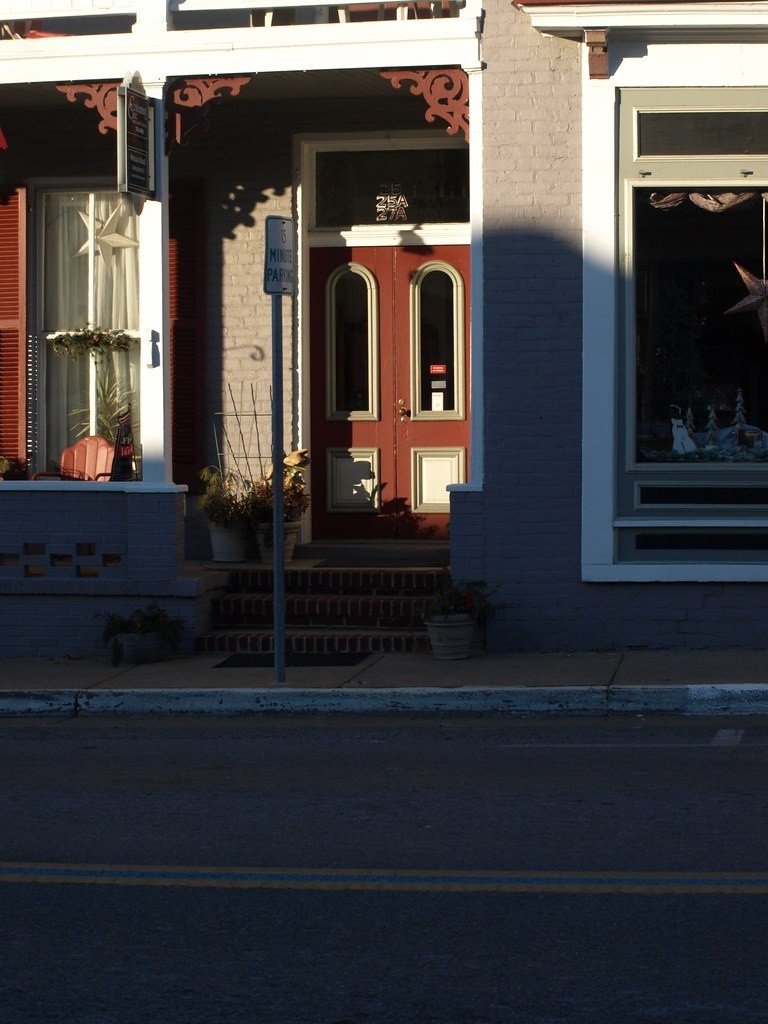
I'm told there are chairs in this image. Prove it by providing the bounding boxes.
[31,435,115,482]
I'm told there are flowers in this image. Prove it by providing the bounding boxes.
[416,579,522,626]
[46,324,141,375]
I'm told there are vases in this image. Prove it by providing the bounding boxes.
[425,612,472,660]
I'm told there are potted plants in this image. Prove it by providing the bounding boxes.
[195,464,312,564]
